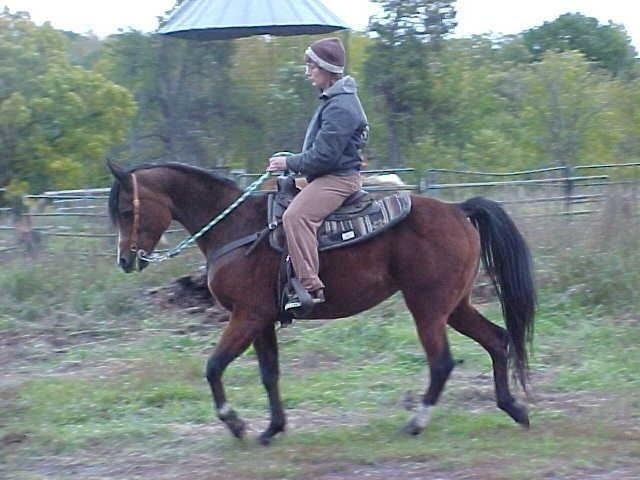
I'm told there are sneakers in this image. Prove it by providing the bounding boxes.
[285,287,327,311]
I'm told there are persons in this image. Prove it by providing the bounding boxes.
[267,38,369,311]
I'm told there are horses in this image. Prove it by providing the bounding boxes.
[104,159,538,449]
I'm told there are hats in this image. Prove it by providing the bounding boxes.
[304,37,346,74]
